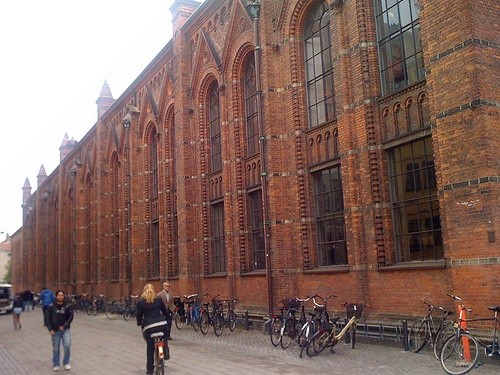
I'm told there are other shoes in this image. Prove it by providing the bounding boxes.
[53,365,60,371]
[168,336,172,340]
[164,351,170,359]
[64,364,71,370]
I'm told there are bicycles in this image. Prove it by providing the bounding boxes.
[137,322,168,375]
[173,293,237,337]
[269,294,369,357]
[407,301,458,362]
[63,294,139,321]
[437,294,500,375]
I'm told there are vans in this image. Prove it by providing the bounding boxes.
[0,284,14,313]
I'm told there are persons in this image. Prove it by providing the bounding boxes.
[136,284,171,375]
[12,295,23,330]
[44,290,74,370]
[157,281,174,341]
[39,287,54,325]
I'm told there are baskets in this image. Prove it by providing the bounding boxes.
[284,297,301,309]
[347,303,363,319]
